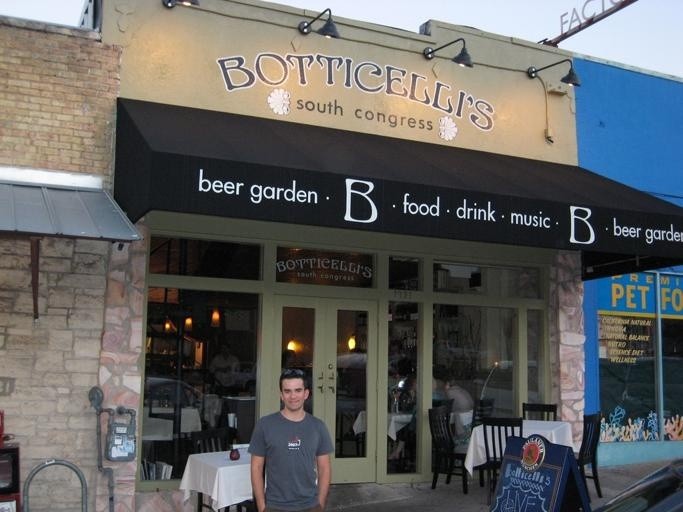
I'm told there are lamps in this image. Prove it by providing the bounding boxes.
[162,290,223,334]
[529,59,581,85]
[163,1,200,9]
[298,9,342,38]
[423,38,474,68]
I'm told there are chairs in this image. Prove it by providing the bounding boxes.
[572,412,603,503]
[427,407,484,494]
[481,416,524,506]
[192,426,236,455]
[522,402,558,420]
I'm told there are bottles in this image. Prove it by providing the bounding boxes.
[230,449,240,460]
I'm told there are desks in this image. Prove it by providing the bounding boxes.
[465,420,576,478]
[180,449,320,510]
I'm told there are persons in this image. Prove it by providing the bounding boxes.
[386,377,443,461]
[440,374,475,438]
[249,367,335,510]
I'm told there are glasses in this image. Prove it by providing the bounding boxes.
[281,368,303,376]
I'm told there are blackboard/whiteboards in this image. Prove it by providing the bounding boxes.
[489,436,572,512]
